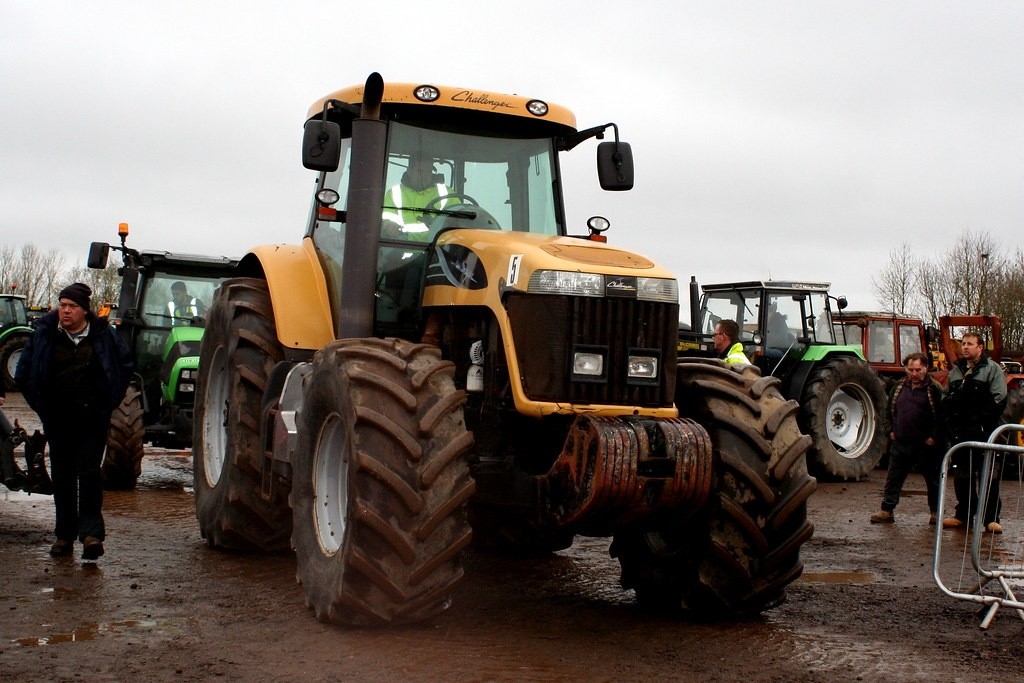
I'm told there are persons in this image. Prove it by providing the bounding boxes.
[16,283,128,561]
[767,302,788,347]
[162,281,208,327]
[712,319,751,364]
[946,332,1008,534]
[381,149,463,280]
[869,353,946,526]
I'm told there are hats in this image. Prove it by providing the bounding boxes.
[58,283,92,313]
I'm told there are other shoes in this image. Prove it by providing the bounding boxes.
[50,538,75,557]
[81,535,104,561]
[930,512,937,524]
[986,521,1003,534]
[870,509,895,524]
[943,516,973,529]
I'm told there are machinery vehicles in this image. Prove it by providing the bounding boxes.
[0,282,53,393]
[689,275,889,484]
[192,70,819,629]
[818,312,1023,482]
[88,222,242,492]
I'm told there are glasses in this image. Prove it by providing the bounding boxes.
[710,333,729,340]
